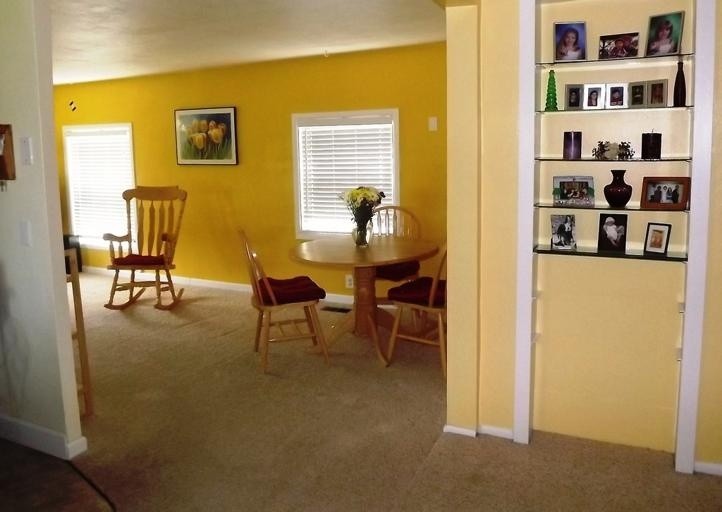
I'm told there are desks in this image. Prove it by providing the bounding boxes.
[287,236,440,368]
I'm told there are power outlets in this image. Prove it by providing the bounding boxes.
[345,274,354,289]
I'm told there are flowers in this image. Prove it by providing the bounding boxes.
[592,140,637,163]
[338,185,386,244]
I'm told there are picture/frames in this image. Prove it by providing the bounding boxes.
[552,173,597,208]
[642,221,673,259]
[173,105,240,167]
[641,175,692,212]
[552,9,688,109]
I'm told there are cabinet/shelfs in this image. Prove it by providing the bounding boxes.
[532,47,695,262]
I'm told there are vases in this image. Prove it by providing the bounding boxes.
[351,223,373,248]
[604,169,633,210]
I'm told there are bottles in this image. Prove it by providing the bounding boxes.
[544,68,559,111]
[673,57,687,106]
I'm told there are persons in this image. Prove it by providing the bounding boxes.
[556,20,675,110]
[554,181,680,255]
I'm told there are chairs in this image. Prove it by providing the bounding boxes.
[236,228,332,372]
[103,184,188,311]
[370,204,423,330]
[386,239,447,379]
